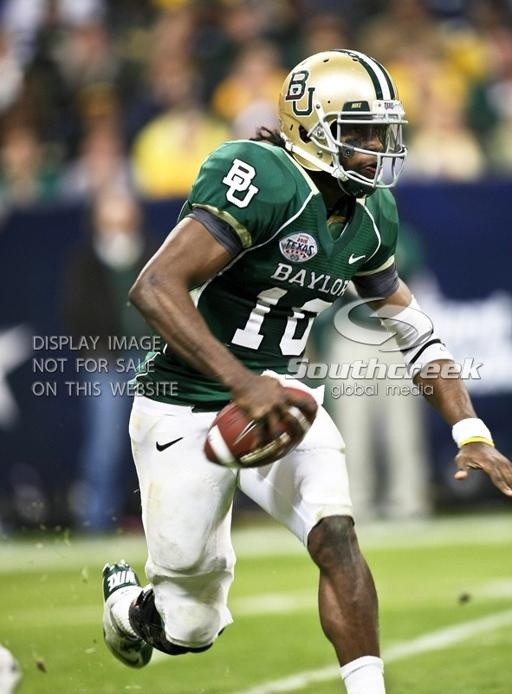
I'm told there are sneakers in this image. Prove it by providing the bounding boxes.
[100,559,153,670]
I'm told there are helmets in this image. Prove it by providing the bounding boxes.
[279,48,407,173]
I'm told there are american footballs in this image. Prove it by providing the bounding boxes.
[204,387,317,468]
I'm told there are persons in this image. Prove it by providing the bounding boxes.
[103,49,512,694]
